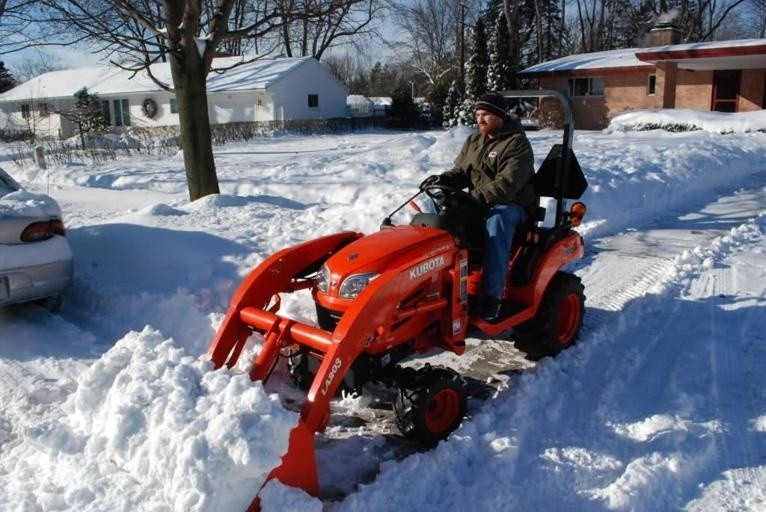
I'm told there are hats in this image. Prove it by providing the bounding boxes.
[475,92,508,118]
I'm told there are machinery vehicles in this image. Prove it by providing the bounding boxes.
[197,85,591,509]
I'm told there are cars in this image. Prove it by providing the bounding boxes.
[0,166,77,317]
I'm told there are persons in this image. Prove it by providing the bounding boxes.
[416,92,539,323]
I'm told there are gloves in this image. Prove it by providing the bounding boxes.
[420,175,444,191]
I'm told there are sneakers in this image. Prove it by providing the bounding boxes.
[480,297,503,322]
